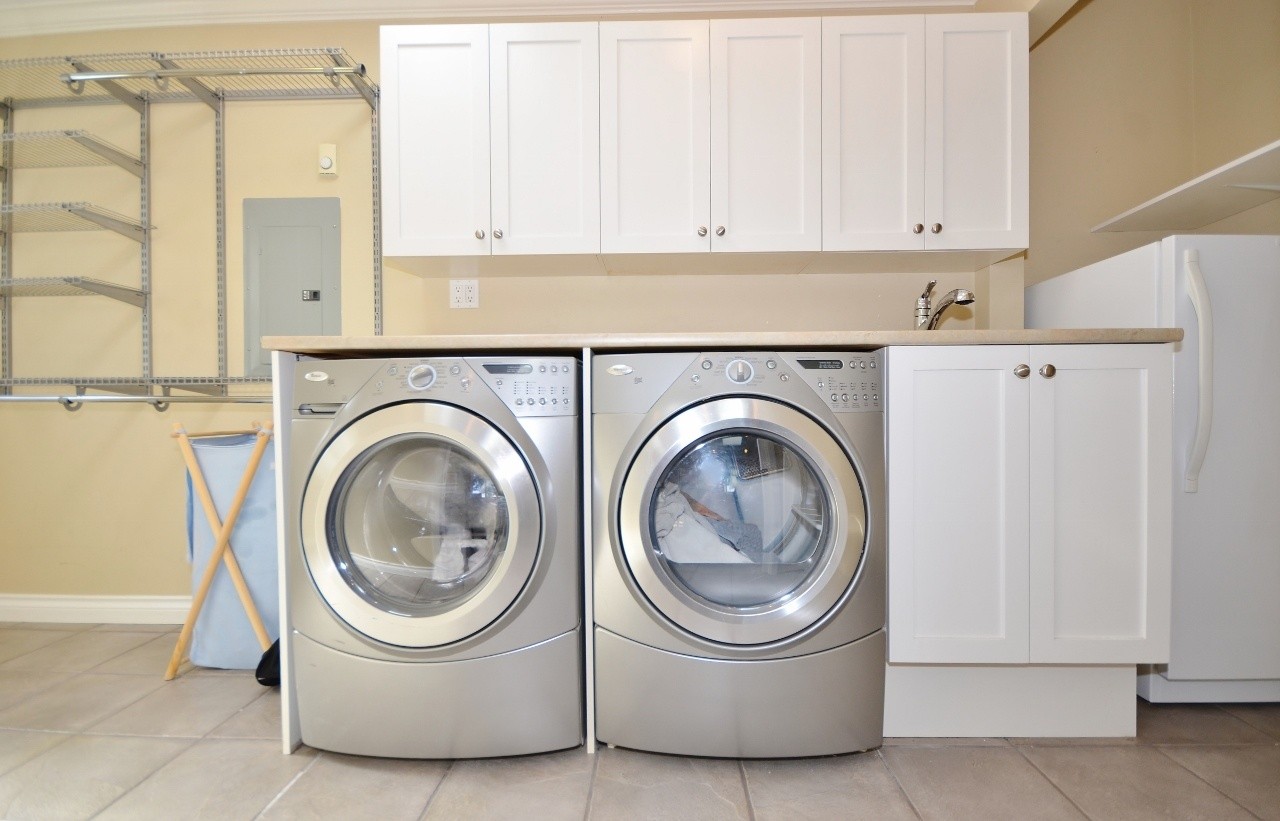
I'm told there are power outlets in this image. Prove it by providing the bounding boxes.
[449,280,479,308]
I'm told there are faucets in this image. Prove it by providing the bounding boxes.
[914,279,976,330]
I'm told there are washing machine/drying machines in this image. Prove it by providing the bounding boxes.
[258,330,891,760]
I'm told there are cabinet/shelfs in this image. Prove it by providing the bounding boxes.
[885,346,1174,741]
[0,41,387,413]
[374,1,1037,274]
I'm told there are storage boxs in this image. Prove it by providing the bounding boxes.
[163,433,281,683]
